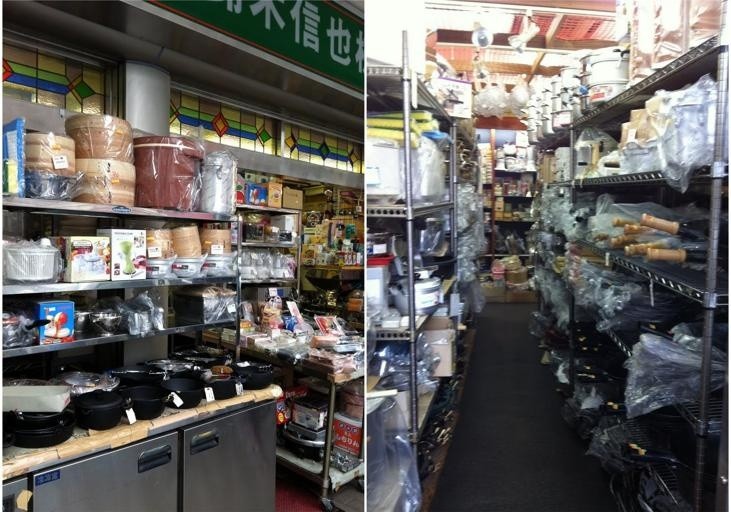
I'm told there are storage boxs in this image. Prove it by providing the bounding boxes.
[257,181,304,209]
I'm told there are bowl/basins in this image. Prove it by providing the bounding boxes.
[74,311,124,337]
[147,257,233,279]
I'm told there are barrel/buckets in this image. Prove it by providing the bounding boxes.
[166,225,201,257]
[200,222,233,257]
[147,227,175,260]
[65,115,134,164]
[22,128,79,178]
[71,158,134,206]
[131,136,205,208]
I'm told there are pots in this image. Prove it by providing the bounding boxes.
[518,45,630,146]
[389,273,442,314]
[2,346,275,447]
[3,310,51,349]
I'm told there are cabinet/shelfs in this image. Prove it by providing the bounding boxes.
[367,1,727,512]
[2,192,365,511]
